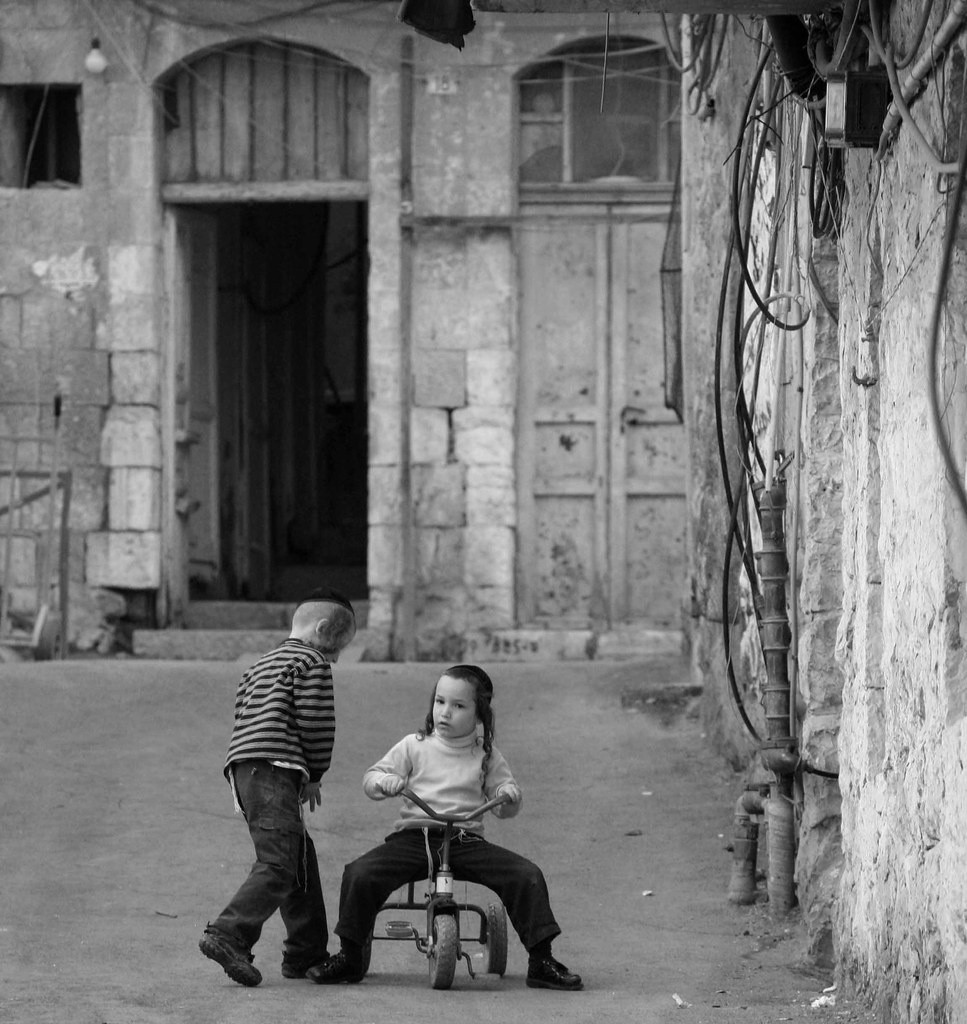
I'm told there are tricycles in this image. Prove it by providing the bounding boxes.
[339,787,513,992]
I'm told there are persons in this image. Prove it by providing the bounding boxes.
[306,665,584,990]
[199,584,355,987]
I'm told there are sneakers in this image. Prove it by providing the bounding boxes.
[525,956,583,992]
[199,931,263,987]
[306,951,364,985]
[281,946,331,978]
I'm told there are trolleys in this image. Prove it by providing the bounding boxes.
[0,393,76,663]
